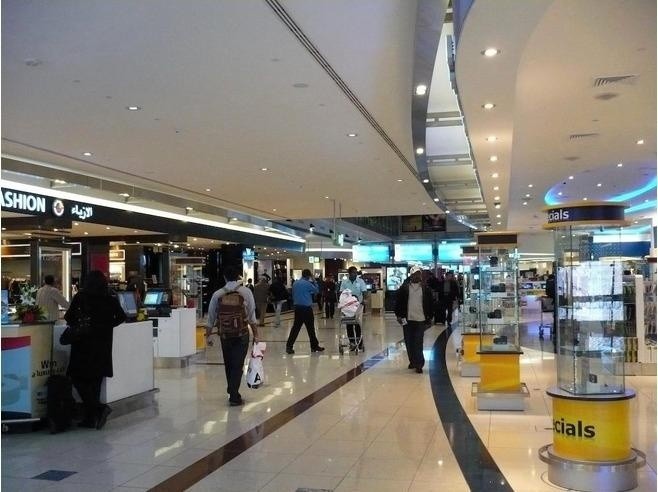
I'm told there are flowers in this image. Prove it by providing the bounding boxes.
[7,278,50,320]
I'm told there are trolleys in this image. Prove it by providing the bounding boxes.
[336,295,366,355]
[538,295,554,338]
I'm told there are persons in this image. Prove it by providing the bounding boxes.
[4,275,22,304]
[314,274,339,319]
[546,274,554,299]
[61,270,126,430]
[393,266,434,374]
[246,276,290,326]
[421,270,464,327]
[339,266,367,351]
[203,266,260,408]
[285,269,325,354]
[35,275,70,324]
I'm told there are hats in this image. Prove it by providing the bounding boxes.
[410,266,422,277]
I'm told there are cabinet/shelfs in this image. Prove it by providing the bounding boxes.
[540,200,640,492]
[460,244,497,377]
[470,233,529,411]
[170,254,206,350]
[621,273,645,364]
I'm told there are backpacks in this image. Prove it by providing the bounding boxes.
[215,285,249,339]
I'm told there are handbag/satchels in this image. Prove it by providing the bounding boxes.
[246,341,267,390]
[59,290,93,346]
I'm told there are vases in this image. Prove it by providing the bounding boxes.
[22,310,38,323]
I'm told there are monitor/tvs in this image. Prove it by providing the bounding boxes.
[142,291,164,306]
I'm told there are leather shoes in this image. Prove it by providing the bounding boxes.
[311,346,325,352]
[286,348,294,354]
[77,403,113,430]
[408,363,415,369]
[416,367,423,373]
[229,392,243,406]
